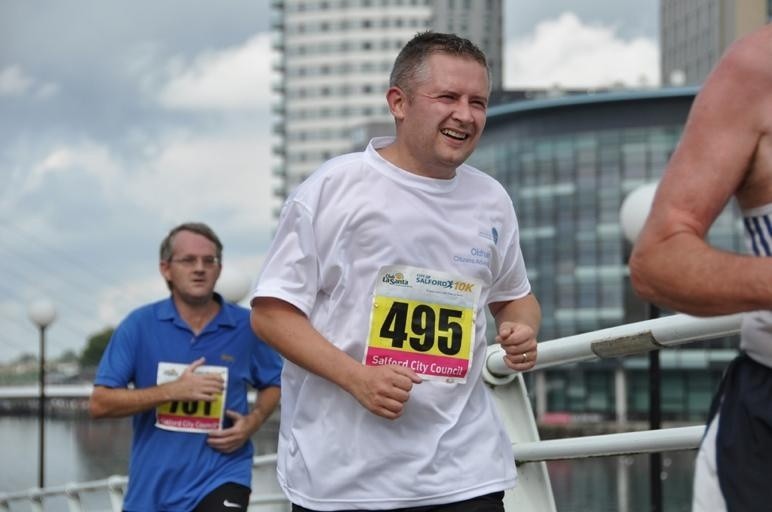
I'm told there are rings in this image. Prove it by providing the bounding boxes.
[523,352,528,362]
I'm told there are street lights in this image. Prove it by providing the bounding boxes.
[620,181,662,512]
[26,295,58,489]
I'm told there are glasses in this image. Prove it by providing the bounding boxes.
[168,255,223,268]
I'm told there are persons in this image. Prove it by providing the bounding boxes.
[249,31,544,511]
[626,20,772,511]
[89,221,283,512]
